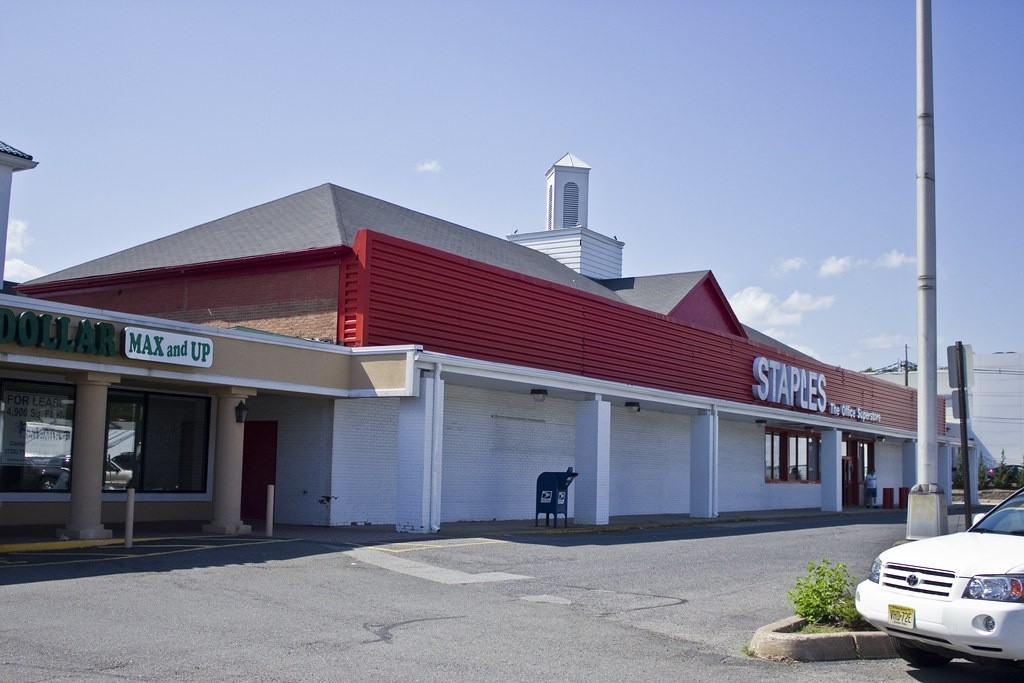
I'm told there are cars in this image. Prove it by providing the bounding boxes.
[38,454,134,492]
[853,485,1023,671]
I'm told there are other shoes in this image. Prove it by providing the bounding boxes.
[867,505,869,508]
[874,506,879,509]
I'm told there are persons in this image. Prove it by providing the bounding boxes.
[864,467,877,509]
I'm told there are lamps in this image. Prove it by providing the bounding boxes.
[842,432,885,442]
[234,399,249,424]
[530,389,548,403]
[624,401,640,412]
[755,420,767,428]
[805,426,815,433]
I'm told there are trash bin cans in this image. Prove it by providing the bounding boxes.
[535,467,579,529]
